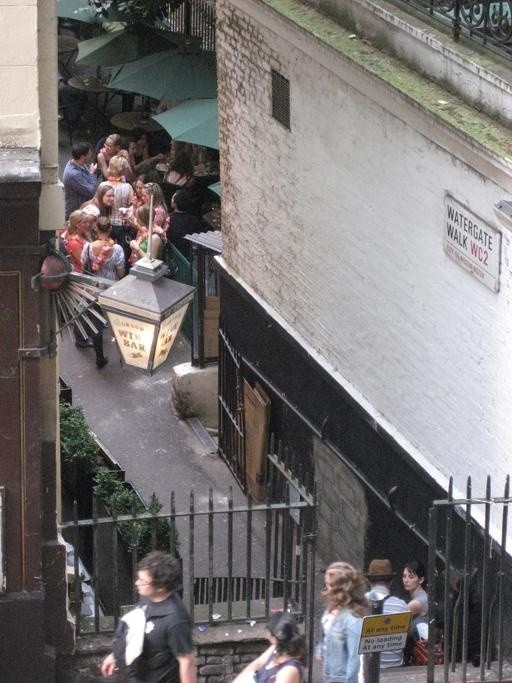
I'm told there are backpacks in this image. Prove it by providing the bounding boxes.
[367,593,393,616]
[112,596,185,683]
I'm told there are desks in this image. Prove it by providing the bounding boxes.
[111,110,165,132]
[58,35,81,75]
[67,73,135,128]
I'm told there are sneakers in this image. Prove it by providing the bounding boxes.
[75,340,108,368]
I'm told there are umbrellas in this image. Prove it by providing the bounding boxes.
[56,0,221,202]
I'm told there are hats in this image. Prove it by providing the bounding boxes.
[363,558,399,578]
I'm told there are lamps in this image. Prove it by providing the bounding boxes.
[31,194,198,376]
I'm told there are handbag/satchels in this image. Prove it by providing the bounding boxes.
[410,638,445,666]
[82,243,93,276]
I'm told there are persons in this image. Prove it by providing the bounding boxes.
[357,558,412,683]
[100,549,197,683]
[402,558,431,661]
[60,126,218,370]
[232,612,304,682]
[313,561,368,682]
